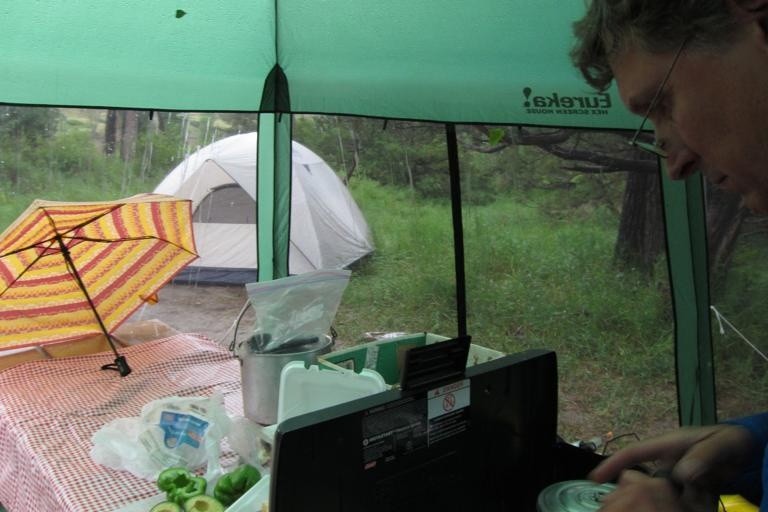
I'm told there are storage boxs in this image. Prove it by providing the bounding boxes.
[317,332,507,389]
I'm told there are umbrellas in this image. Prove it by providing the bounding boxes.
[0,193,201,376]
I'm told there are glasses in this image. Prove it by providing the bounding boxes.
[628,27,699,158]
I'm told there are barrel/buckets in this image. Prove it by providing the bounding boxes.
[228,297,335,426]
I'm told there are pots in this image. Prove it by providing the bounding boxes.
[233,326,338,427]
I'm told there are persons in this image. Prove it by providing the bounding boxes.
[566,2,767,512]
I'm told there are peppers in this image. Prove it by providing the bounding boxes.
[150,468,223,512]
[214,465,261,504]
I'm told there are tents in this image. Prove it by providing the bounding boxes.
[148,131,377,285]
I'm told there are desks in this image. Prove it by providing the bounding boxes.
[0,319,247,511]
